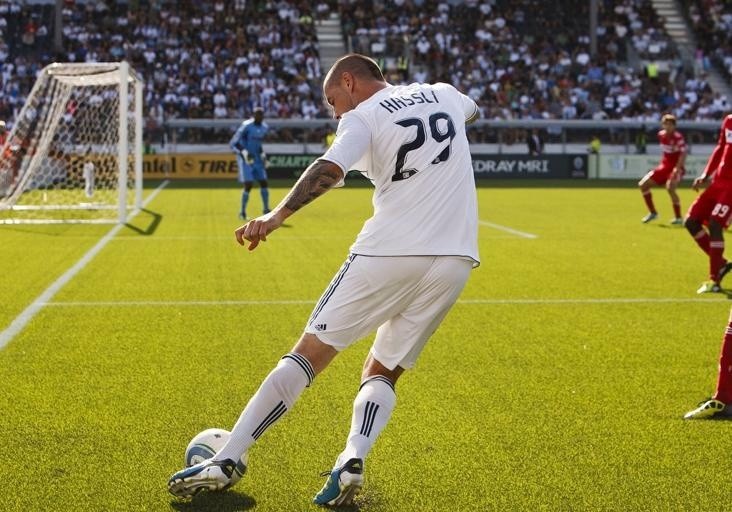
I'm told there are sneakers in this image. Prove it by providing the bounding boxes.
[314,458,364,506]
[641,213,656,223]
[671,218,682,224]
[681,399,732,420]
[696,280,721,294]
[166,457,237,498]
[720,262,732,279]
[263,209,271,214]
[239,213,245,219]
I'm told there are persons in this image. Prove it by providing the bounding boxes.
[229,107,271,219]
[166,53,482,506]
[82,158,94,198]
[684,114,732,293]
[637,115,689,223]
[1,1,732,155]
[684,308,732,419]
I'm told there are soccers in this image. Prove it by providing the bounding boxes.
[186,428,247,488]
